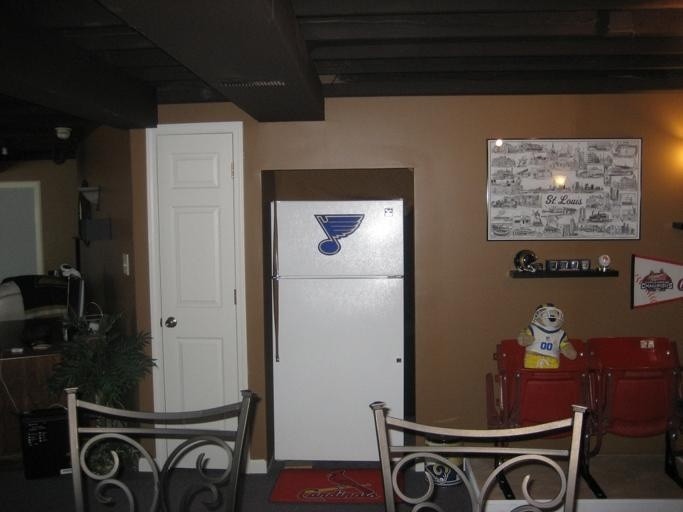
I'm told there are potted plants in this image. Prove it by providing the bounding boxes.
[45,307,159,490]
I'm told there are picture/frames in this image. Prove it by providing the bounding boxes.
[486,138,641,240]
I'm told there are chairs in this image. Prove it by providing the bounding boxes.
[481,337,682,499]
[65,386,252,512]
[370,396,590,511]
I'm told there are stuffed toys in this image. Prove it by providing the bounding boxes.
[517,301,579,370]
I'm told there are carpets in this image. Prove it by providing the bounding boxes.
[269,469,402,505]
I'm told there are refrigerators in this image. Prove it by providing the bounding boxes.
[269,198,406,465]
